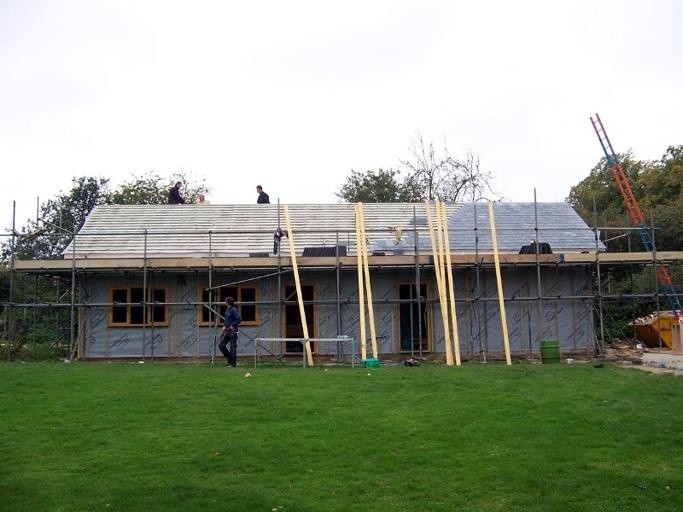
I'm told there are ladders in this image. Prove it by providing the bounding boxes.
[589,113,682,316]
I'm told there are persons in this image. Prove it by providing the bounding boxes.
[196,194,210,204]
[219,296,242,367]
[168,181,189,203]
[256,185,269,203]
